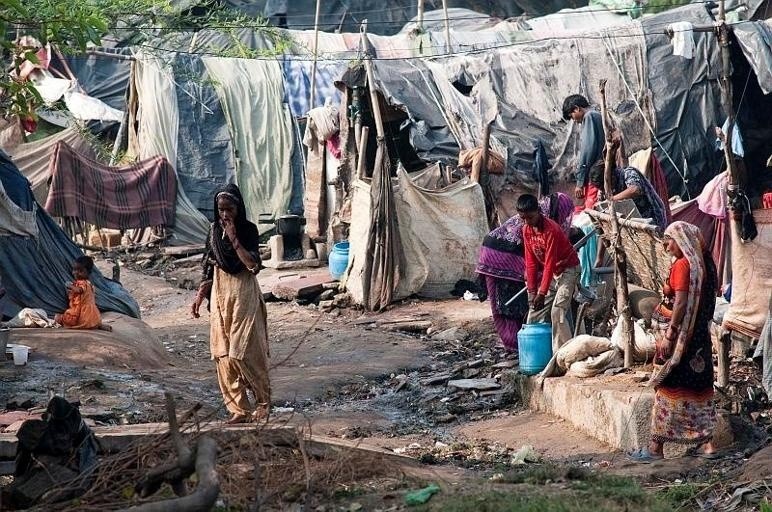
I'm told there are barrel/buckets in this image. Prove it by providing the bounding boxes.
[329,241,349,277]
[12,346,28,365]
[518,323,552,374]
[0,329,11,361]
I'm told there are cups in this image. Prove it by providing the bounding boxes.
[13,346,28,366]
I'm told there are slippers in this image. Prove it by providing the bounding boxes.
[686,447,720,458]
[623,445,664,463]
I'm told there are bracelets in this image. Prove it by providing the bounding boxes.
[664,334,673,341]
[231,237,236,244]
[234,240,240,250]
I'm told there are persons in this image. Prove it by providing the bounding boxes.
[561,94,614,212]
[474,191,575,360]
[189,181,273,426]
[628,220,721,460]
[53,255,114,331]
[587,162,667,269]
[516,193,582,362]
